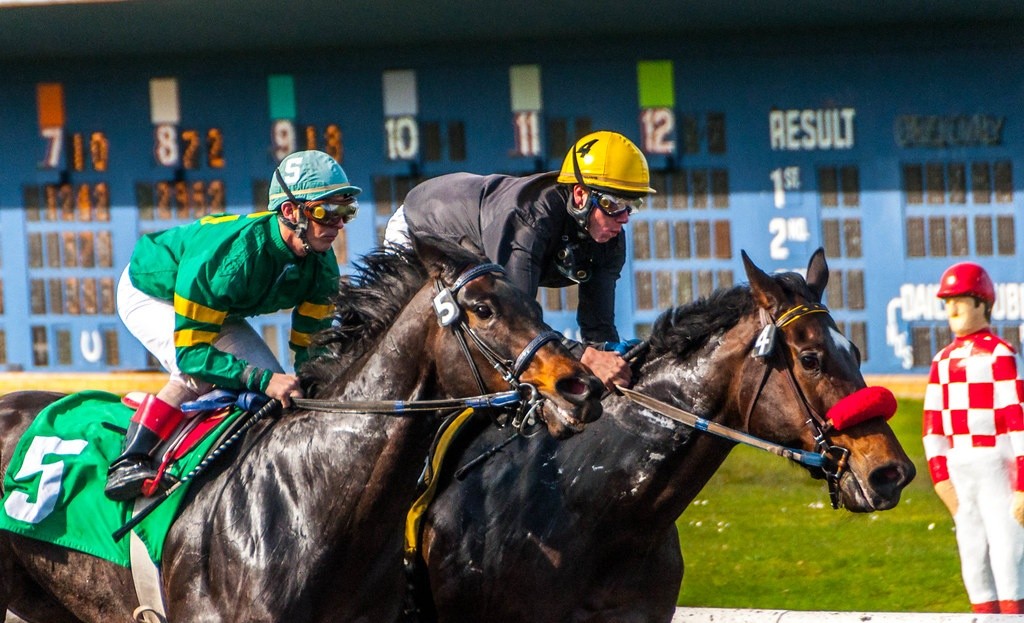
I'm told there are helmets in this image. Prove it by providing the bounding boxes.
[268,150,362,211]
[557,130,657,228]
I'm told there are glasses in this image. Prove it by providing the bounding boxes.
[589,189,643,217]
[303,196,359,226]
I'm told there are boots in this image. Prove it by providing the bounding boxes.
[104,393,180,501]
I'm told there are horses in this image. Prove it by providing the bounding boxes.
[0,225,610,623]
[330,246,918,623]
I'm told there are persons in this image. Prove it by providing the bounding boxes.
[102,149,364,503]
[382,130,658,391]
[918,257,1023,614]
